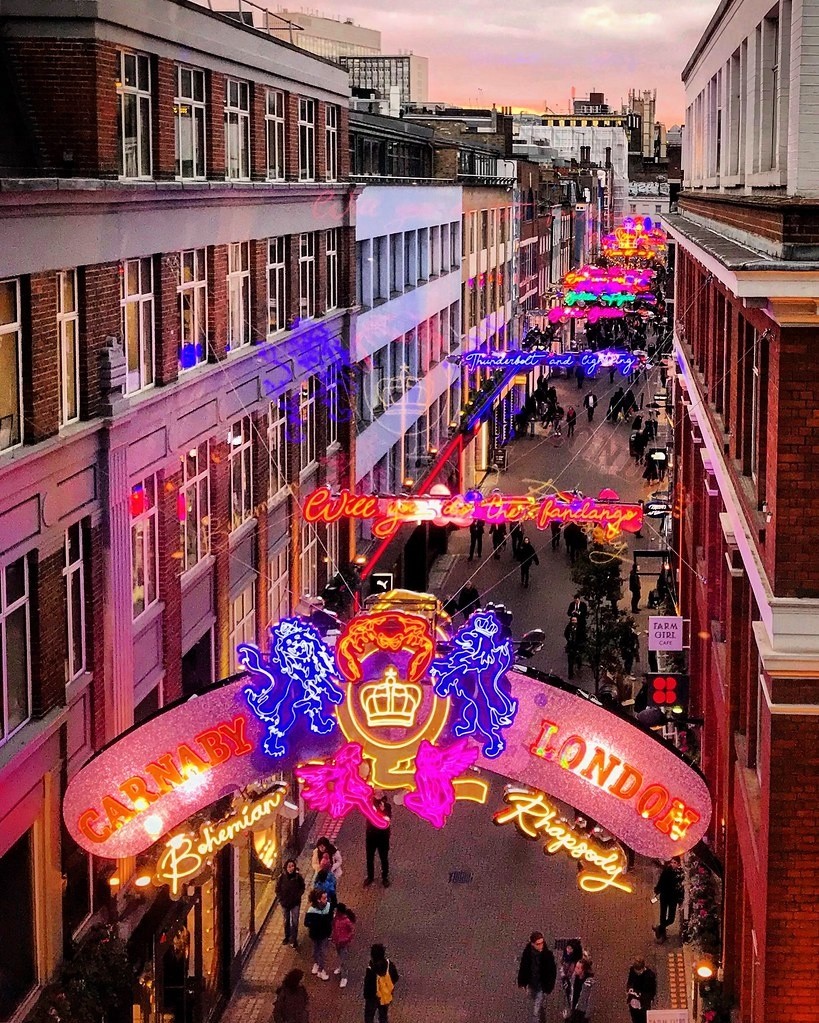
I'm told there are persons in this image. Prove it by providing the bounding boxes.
[560,939,596,1023]
[363,943,399,1023]
[625,958,658,1023]
[517,930,557,1023]
[443,581,480,619]
[467,519,528,561]
[518,536,539,588]
[566,594,589,624]
[521,268,673,487]
[629,564,645,615]
[271,969,311,1023]
[649,856,681,943]
[700,967,739,1023]
[565,614,586,680]
[277,858,304,950]
[311,837,343,903]
[620,617,640,674]
[304,886,355,989]
[550,522,561,549]
[363,790,391,888]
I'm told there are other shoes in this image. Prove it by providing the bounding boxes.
[364,876,374,885]
[381,879,390,887]
[293,939,298,948]
[311,963,330,979]
[282,937,290,944]
[654,926,666,942]
[339,978,348,988]
[334,966,342,974]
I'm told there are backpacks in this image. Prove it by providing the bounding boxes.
[370,960,395,1005]
